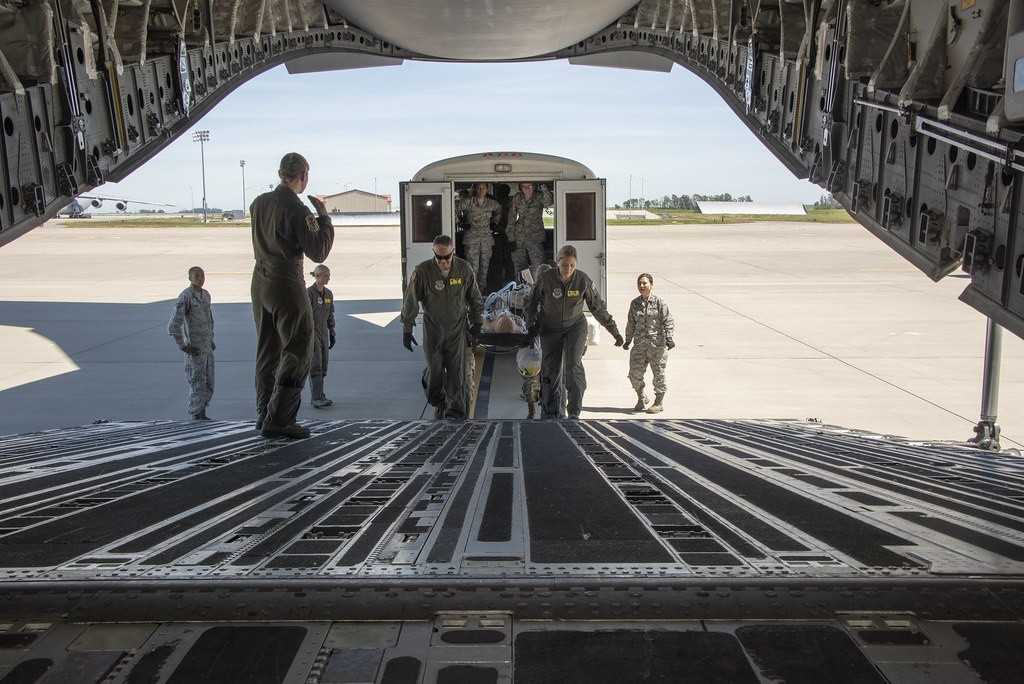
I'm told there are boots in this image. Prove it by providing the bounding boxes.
[635,387,649,410]
[646,392,665,413]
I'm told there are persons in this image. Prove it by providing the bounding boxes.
[400,235,483,421]
[248,152,334,438]
[481,311,525,334]
[524,245,624,419]
[455,182,553,294]
[306,265,336,408]
[623,273,675,414]
[168,267,216,420]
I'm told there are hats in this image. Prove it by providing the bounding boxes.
[279,152,309,177]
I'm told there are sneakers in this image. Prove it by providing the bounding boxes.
[260,420,311,439]
[255,418,265,430]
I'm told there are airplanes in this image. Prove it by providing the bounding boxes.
[51,194,179,220]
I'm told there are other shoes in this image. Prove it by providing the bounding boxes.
[434,407,446,418]
[312,394,333,407]
[559,414,568,420]
[193,409,211,421]
[444,409,465,420]
[568,414,579,420]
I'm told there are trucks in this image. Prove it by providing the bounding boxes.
[398,152,607,314]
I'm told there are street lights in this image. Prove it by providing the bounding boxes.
[192,130,210,222]
[629,175,634,219]
[240,160,246,216]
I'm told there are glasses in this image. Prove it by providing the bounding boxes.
[433,249,454,260]
[521,182,535,189]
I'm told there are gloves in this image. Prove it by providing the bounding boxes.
[510,241,517,252]
[469,324,481,335]
[490,222,498,231]
[182,346,200,356]
[664,337,675,350]
[211,341,217,351]
[402,332,418,353]
[328,336,335,349]
[528,324,538,337]
[458,220,470,230]
[614,334,624,347]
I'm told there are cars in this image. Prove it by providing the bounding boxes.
[221,210,235,220]
